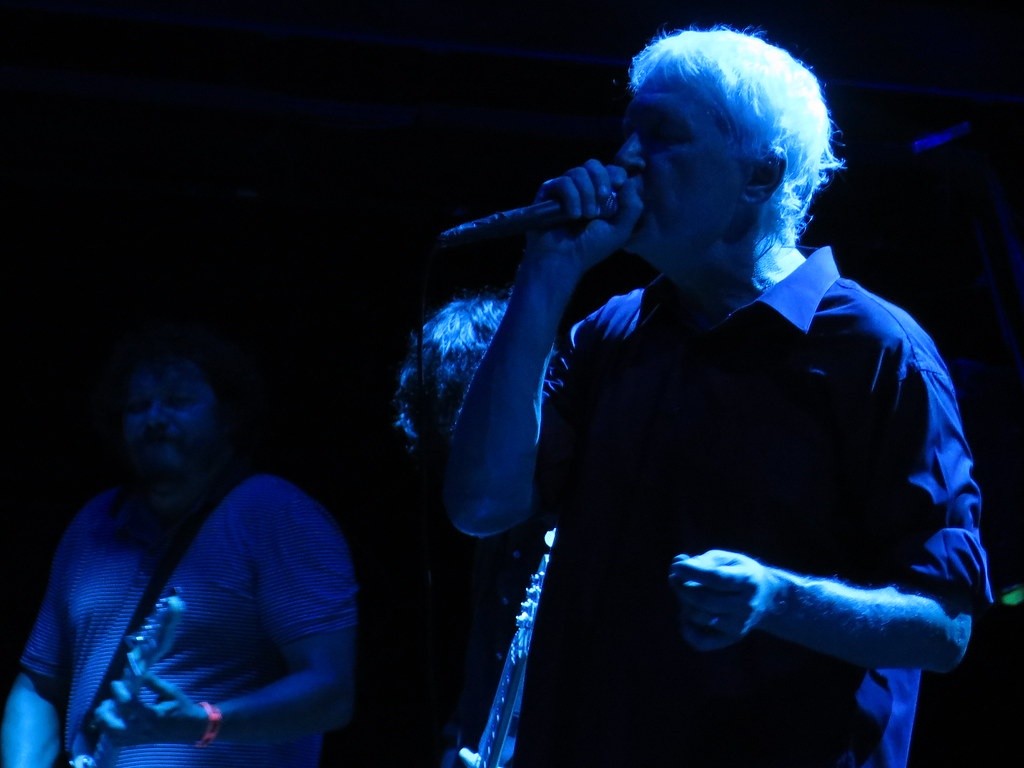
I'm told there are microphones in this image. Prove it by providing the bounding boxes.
[439,184,619,249]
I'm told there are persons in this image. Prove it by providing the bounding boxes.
[0,327,365,768]
[436,24,996,768]
[398,293,568,486]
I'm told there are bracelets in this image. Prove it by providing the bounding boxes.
[198,699,222,745]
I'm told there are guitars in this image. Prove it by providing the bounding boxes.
[72,590,185,768]
[477,524,559,767]
